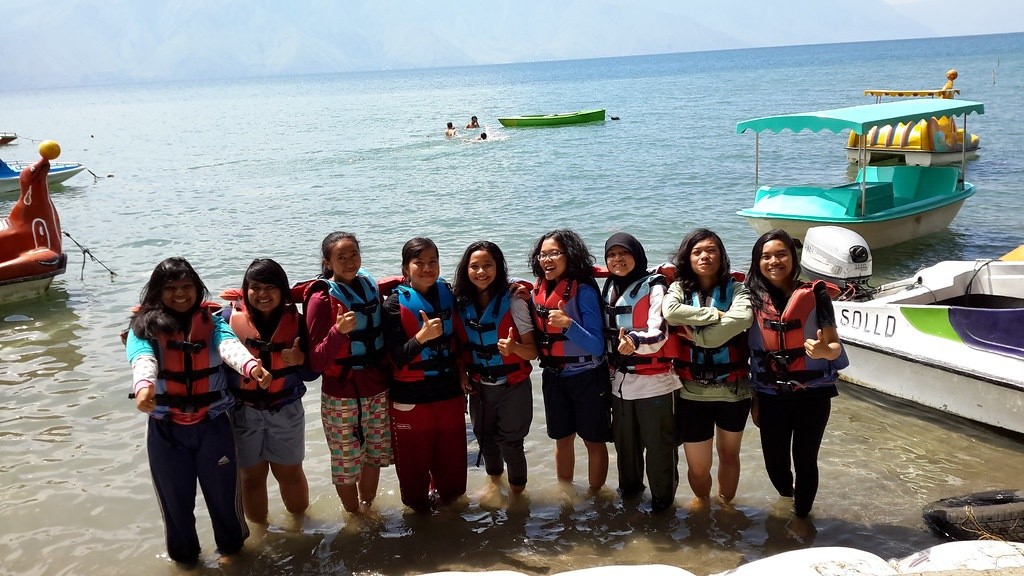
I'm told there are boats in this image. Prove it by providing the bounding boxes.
[0,139,69,306]
[0,134,17,145]
[498,108,606,128]
[830,257,1024,439]
[735,98,984,256]
[843,70,983,168]
[0,153,86,193]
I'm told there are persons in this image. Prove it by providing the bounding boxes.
[126,256,273,564]
[660,227,757,505]
[600,233,684,509]
[509,229,613,488]
[452,240,539,494]
[220,257,321,524]
[379,236,473,510]
[300,232,395,515]
[445,122,456,136]
[477,133,488,141]
[466,116,480,128]
[745,227,850,515]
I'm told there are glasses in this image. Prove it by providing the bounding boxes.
[536,249,565,263]
